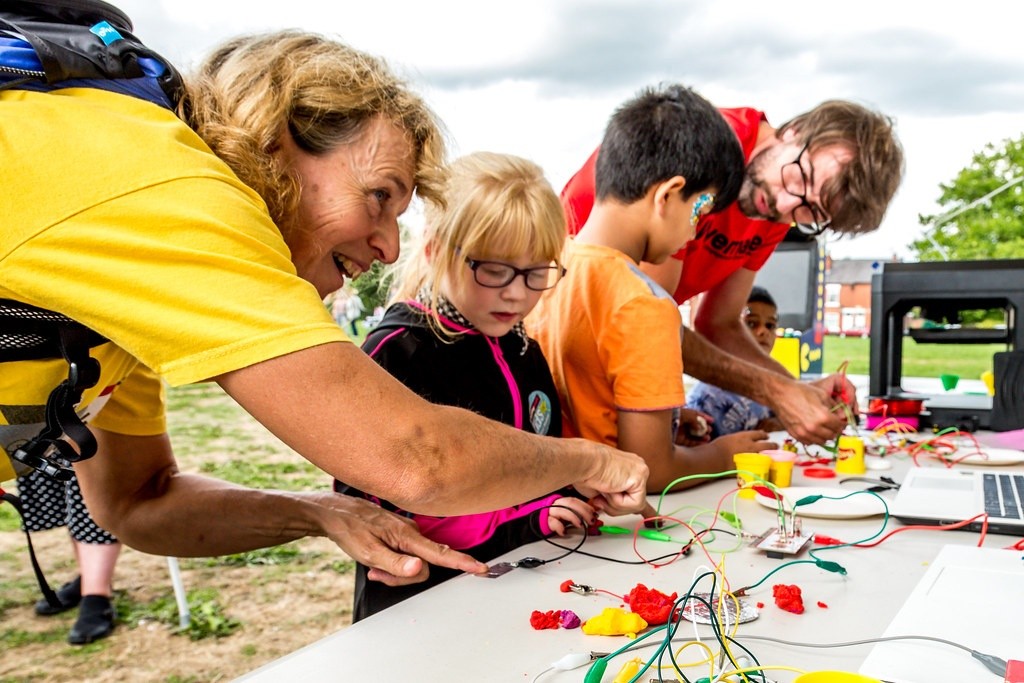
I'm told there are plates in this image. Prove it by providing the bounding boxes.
[933,446,1023,467]
[753,484,890,520]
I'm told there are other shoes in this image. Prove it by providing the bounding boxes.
[35,575,81,616]
[69,594,115,644]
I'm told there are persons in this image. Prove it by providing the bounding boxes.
[331,84,904,625]
[0,29,663,584]
[16,452,121,645]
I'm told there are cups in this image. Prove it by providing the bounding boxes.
[835,436,867,475]
[760,450,795,488]
[733,453,770,500]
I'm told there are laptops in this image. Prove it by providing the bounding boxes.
[890,468,1024,536]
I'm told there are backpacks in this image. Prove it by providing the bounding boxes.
[0,0,227,470]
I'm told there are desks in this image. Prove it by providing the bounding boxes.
[229,374,1024,683]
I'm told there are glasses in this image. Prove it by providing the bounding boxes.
[456,245,567,292]
[781,137,831,236]
